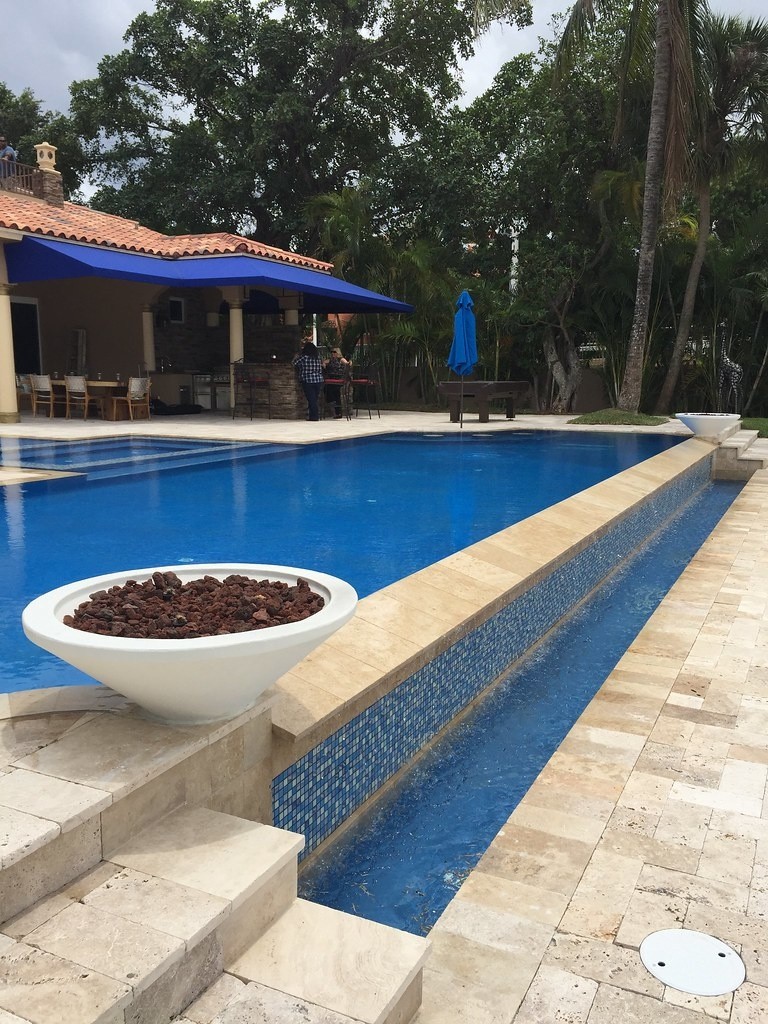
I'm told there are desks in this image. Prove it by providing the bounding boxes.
[21,379,127,419]
[437,380,529,422]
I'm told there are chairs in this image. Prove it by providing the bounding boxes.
[63,374,105,422]
[320,364,352,421]
[29,374,72,419]
[233,363,271,421]
[350,352,380,419]
[15,373,34,415]
[112,376,151,422]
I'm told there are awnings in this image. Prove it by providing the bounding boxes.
[5,236,415,315]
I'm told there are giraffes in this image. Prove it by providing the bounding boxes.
[715,320,746,414]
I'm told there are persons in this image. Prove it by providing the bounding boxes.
[0,137,16,178]
[321,348,352,418]
[290,342,324,421]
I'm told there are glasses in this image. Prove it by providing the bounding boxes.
[330,350,337,354]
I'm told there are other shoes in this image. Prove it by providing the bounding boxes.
[306,417,318,421]
[328,401,336,406]
[333,414,342,419]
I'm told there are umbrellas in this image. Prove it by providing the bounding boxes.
[448,290,477,429]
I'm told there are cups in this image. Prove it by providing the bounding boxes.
[54,371,58,379]
[70,372,75,376]
[98,373,102,381]
[117,373,120,381]
